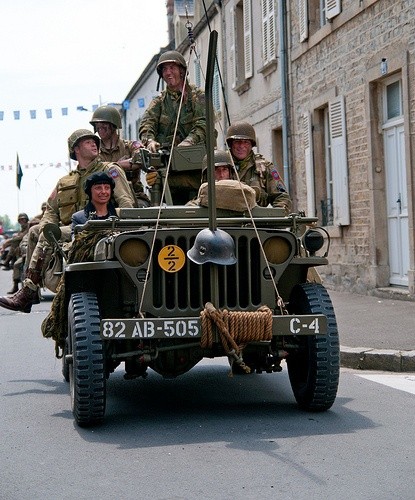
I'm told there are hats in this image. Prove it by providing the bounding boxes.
[83,174,117,191]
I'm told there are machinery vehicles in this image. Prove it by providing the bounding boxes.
[42,29,342,429]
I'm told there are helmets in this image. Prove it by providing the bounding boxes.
[153,51,190,75]
[200,150,236,174]
[15,212,30,221]
[225,124,257,149]
[66,128,100,162]
[184,231,240,267]
[90,106,126,129]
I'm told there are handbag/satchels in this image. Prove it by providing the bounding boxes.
[197,179,263,214]
[41,251,63,293]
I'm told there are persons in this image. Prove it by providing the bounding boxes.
[0,129,134,313]
[71,172,116,240]
[227,121,292,218]
[139,50,218,205]
[185,149,234,206]
[0,213,29,271]
[33,202,46,219]
[7,218,40,294]
[89,105,150,208]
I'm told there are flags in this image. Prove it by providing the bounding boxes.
[16,154,23,189]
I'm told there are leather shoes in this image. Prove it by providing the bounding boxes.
[1,283,36,315]
